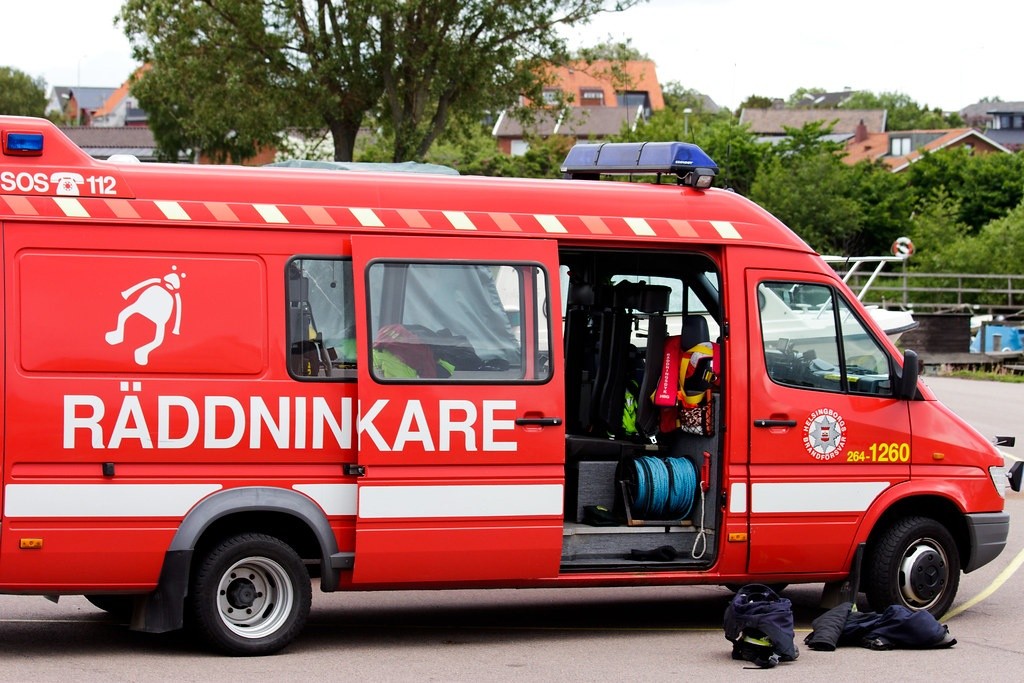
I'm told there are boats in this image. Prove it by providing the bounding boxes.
[493,255,920,375]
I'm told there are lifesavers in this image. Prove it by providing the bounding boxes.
[892,237,912,259]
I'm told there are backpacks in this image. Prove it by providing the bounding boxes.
[724,585,798,668]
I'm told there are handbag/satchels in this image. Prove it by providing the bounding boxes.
[674,387,716,439]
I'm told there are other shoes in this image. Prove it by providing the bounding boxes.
[936,633,957,648]
[864,634,890,650]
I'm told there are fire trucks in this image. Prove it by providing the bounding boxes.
[0,112,1024,659]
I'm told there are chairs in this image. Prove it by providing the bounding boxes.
[681,312,709,351]
[567,275,635,462]
